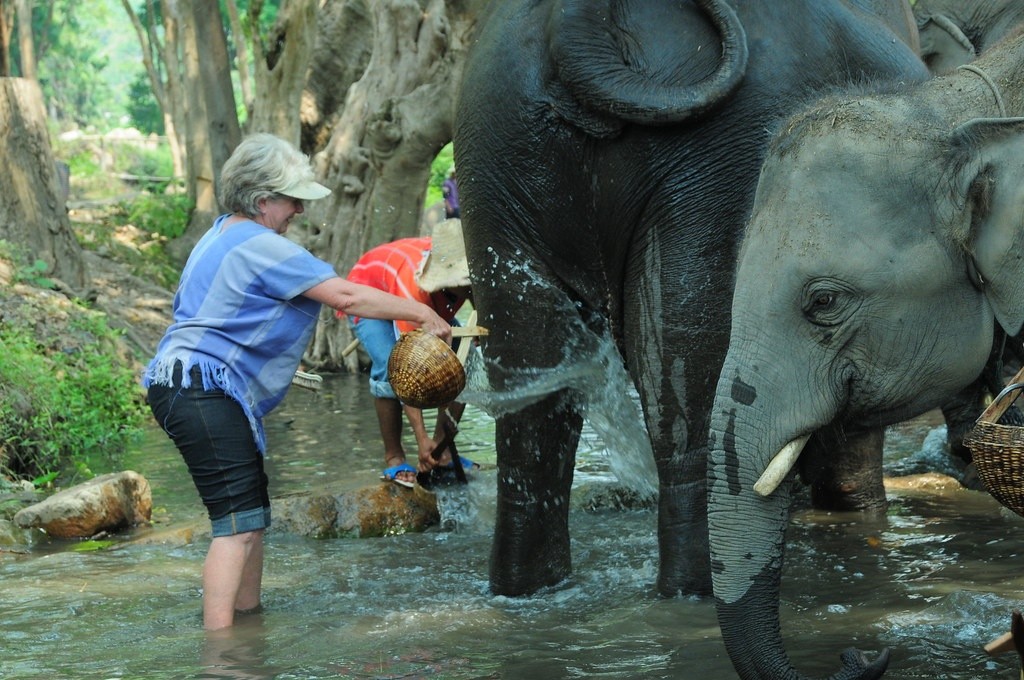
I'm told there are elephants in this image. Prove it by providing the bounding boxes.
[449,0,1024,679]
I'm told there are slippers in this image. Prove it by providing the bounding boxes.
[379,464,418,487]
[448,456,482,472]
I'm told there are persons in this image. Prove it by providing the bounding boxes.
[143,133,450,634]
[350,216,475,489]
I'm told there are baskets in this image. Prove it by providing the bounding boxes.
[961,365,1024,516]
[388,310,489,408]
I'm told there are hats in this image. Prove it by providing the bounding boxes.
[270,181,331,200]
[416,217,472,293]
